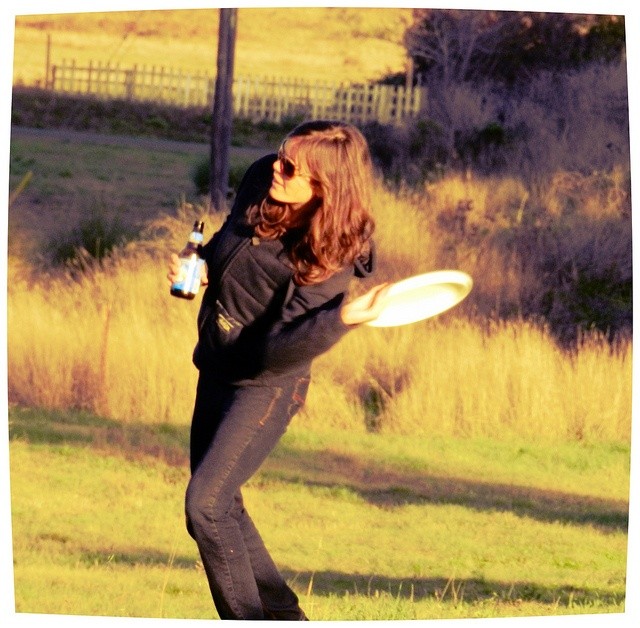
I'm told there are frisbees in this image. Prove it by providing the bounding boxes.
[366,269,473,329]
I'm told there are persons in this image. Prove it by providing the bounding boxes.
[167,119,390,620]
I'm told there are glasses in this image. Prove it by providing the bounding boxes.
[277,150,312,180]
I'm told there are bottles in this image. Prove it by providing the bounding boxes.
[167,214,210,298]
